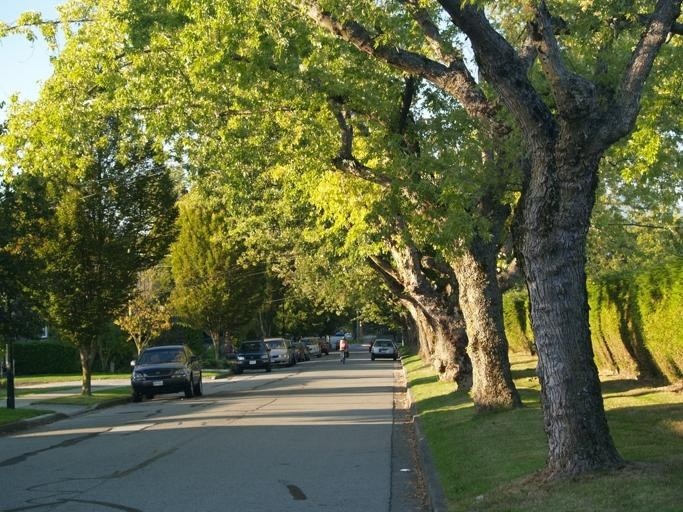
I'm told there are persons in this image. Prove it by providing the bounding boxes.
[335,336,349,366]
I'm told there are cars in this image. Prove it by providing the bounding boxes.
[232,340,273,374]
[129,343,202,400]
[264,330,352,366]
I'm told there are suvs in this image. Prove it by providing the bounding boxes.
[369,337,398,360]
